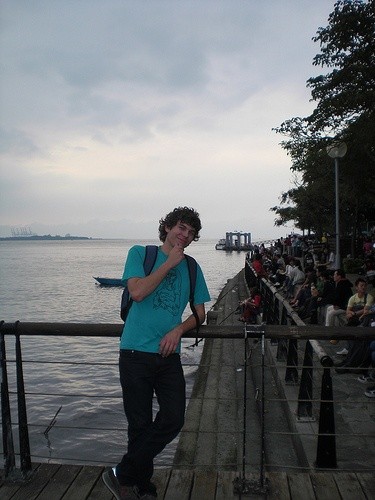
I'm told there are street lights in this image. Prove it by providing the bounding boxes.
[325,138,348,269]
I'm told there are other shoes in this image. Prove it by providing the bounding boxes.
[364,390,375,397]
[357,375,375,383]
[290,300,296,305]
[309,319,319,324]
[292,303,298,308]
[237,316,247,322]
[102,467,157,500]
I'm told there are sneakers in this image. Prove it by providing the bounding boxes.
[337,348,349,354]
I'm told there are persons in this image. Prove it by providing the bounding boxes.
[100,205,212,500]
[234,227,375,400]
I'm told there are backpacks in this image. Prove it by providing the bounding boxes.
[121,246,197,322]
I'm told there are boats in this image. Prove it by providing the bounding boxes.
[92,275,123,286]
